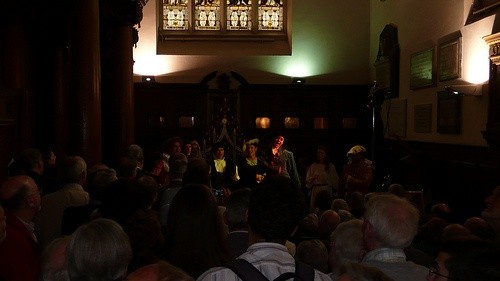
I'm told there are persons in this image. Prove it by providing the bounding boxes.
[0,132,500,281]
